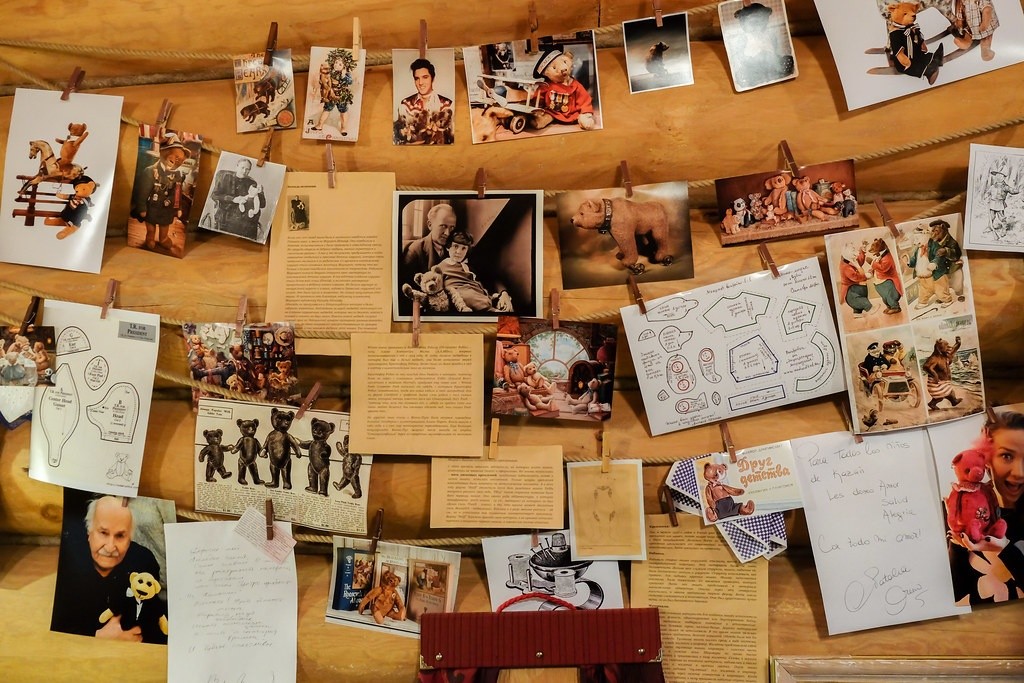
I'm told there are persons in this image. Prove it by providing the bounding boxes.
[211,159,266,240]
[405,204,512,312]
[51,496,168,646]
[311,49,358,136]
[431,232,503,312]
[291,196,308,225]
[949,414,1023,605]
[397,59,452,124]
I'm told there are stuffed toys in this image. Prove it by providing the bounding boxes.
[402,107,454,145]
[402,266,472,312]
[359,570,409,624]
[502,348,601,414]
[187,328,298,406]
[705,463,755,522]
[54,121,90,172]
[1,334,50,381]
[528,43,596,132]
[44,176,97,241]
[98,571,168,636]
[721,173,854,235]
[320,63,340,103]
[733,3,794,87]
[947,450,1007,541]
[887,0,1000,85]
[136,133,193,250]
